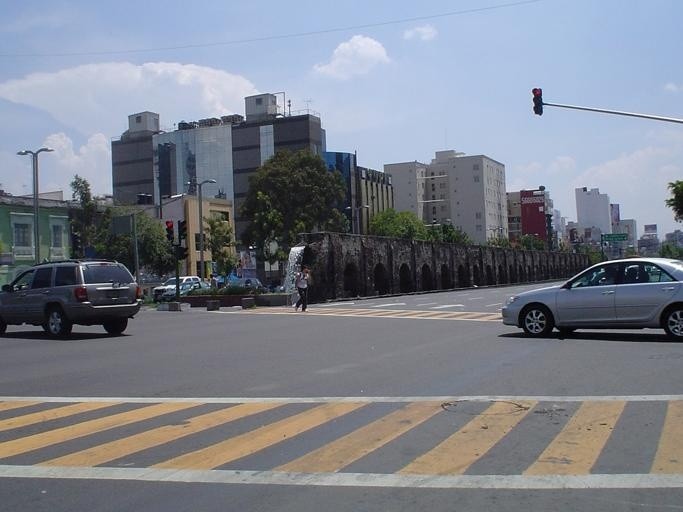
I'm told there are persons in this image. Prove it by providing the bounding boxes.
[602,266,617,284]
[625,268,637,281]
[294,264,310,311]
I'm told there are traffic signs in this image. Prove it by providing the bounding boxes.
[602,234,627,241]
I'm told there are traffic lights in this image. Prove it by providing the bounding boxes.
[165,220,173,244]
[146,195,152,204]
[177,220,186,241]
[137,194,145,204]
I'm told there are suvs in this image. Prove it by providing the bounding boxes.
[0,258,142,339]
[152,275,200,303]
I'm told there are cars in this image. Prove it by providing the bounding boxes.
[501,257,683,342]
[262,283,274,293]
[225,278,262,291]
[161,281,211,302]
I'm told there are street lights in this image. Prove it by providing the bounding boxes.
[531,87,542,116]
[184,179,216,282]
[485,227,503,247]
[344,204,370,235]
[248,239,256,279]
[16,147,54,265]
[421,218,452,240]
[520,233,539,250]
